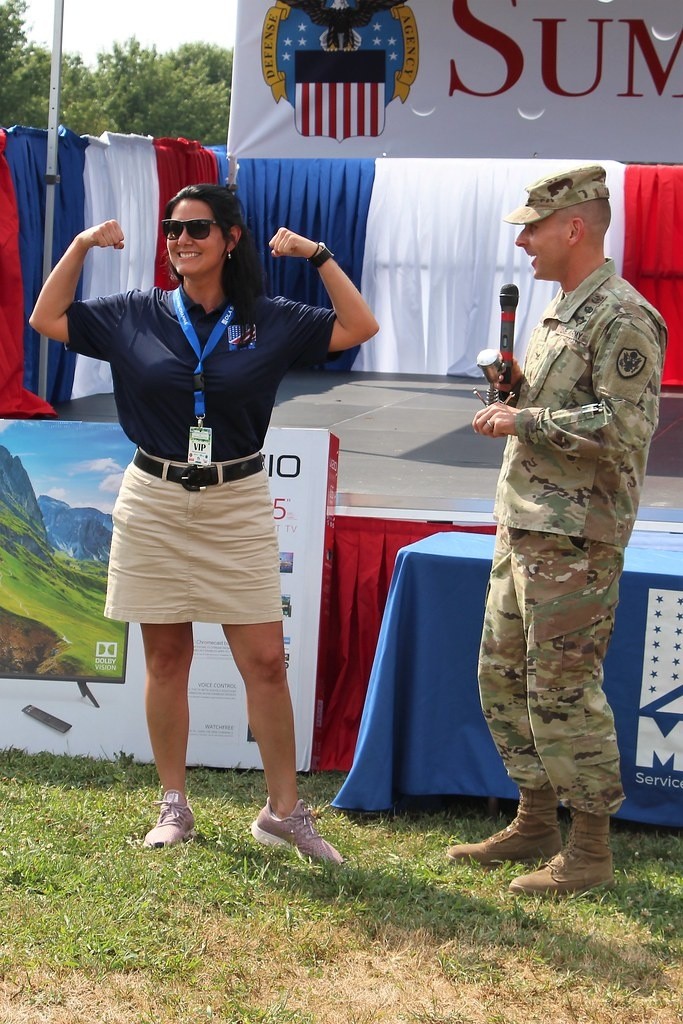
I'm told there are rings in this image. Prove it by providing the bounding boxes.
[486,418,492,428]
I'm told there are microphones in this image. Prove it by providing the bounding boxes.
[499,283,521,387]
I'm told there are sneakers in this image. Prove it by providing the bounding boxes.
[143,791,194,849]
[252,796,344,868]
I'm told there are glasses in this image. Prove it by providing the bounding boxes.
[160,218,218,240]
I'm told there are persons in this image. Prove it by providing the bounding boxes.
[28,184,379,867]
[446,162,667,898]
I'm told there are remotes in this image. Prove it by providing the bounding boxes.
[21,704,72,733]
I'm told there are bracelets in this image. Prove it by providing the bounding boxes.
[307,242,335,267]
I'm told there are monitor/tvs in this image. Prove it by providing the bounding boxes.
[0,420,130,709]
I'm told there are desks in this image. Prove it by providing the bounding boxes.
[332,531,683,829]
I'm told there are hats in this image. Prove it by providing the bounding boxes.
[501,165,609,225]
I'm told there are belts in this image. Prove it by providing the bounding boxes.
[133,448,264,491]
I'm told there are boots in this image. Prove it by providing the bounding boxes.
[510,806,614,899]
[447,788,562,866]
